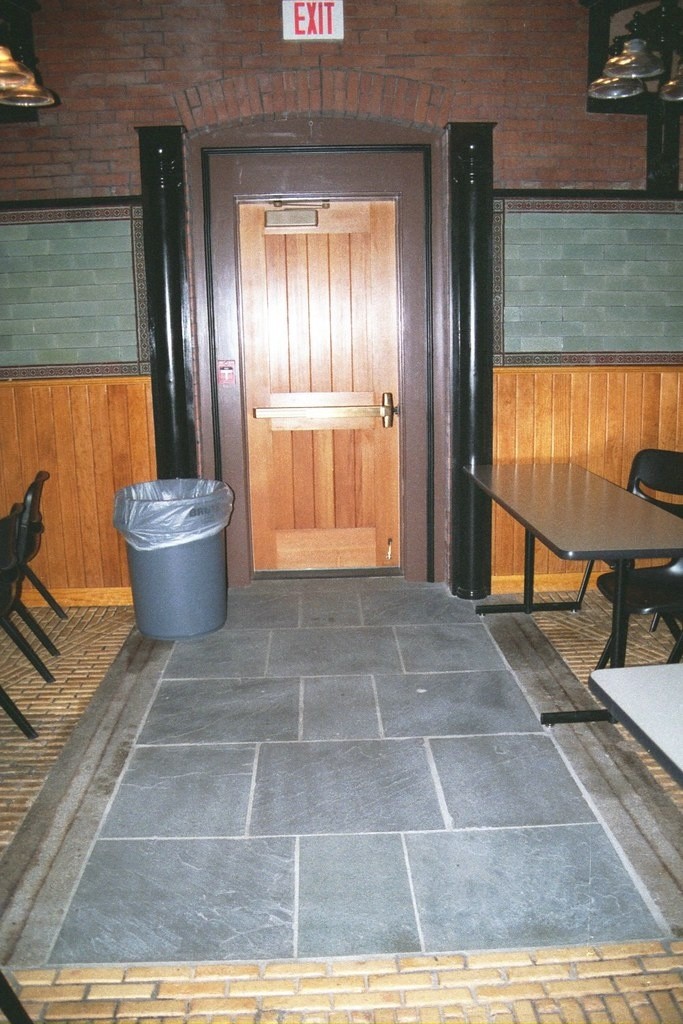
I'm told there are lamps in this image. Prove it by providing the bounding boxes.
[0,0,56,108]
[587,0,683,102]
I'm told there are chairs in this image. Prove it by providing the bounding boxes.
[592,447,683,671]
[0,500,58,742]
[13,470,68,661]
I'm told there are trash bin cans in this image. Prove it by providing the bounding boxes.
[114,476,229,639]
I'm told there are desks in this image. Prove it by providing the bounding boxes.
[460,460,683,729]
[588,664,683,790]
